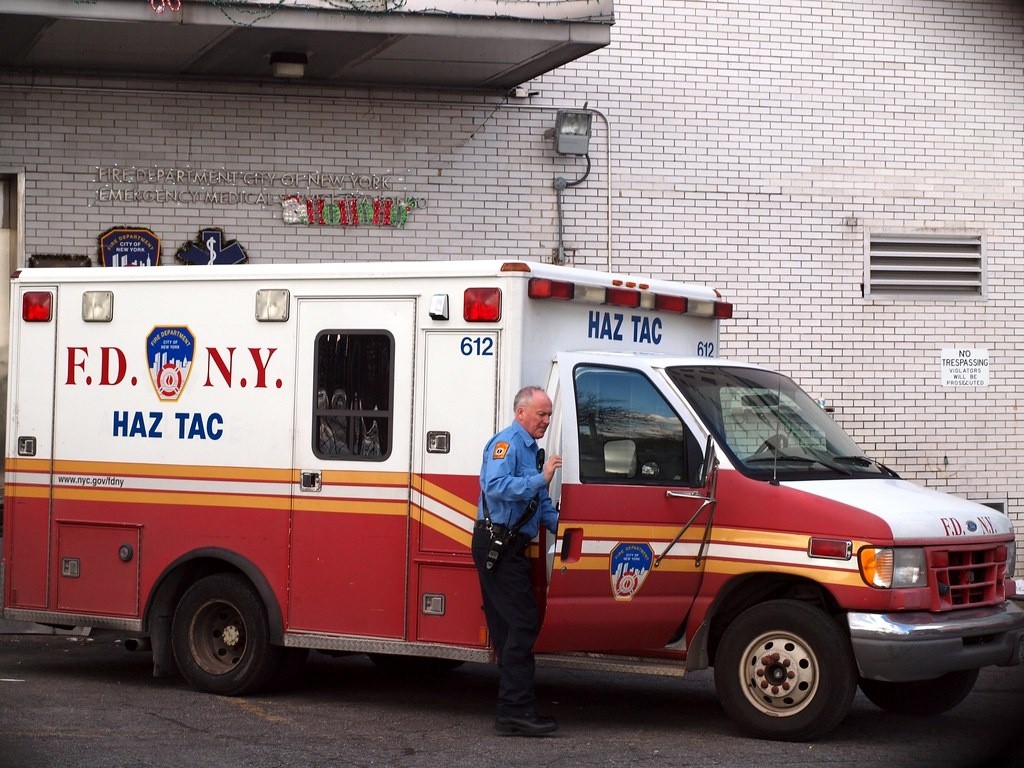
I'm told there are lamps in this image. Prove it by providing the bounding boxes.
[553,107,595,158]
[268,50,309,77]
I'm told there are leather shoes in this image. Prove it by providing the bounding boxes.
[494,706,559,734]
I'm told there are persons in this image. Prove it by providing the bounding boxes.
[471,387,562,735]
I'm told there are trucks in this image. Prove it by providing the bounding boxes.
[2,258,1024,741]
